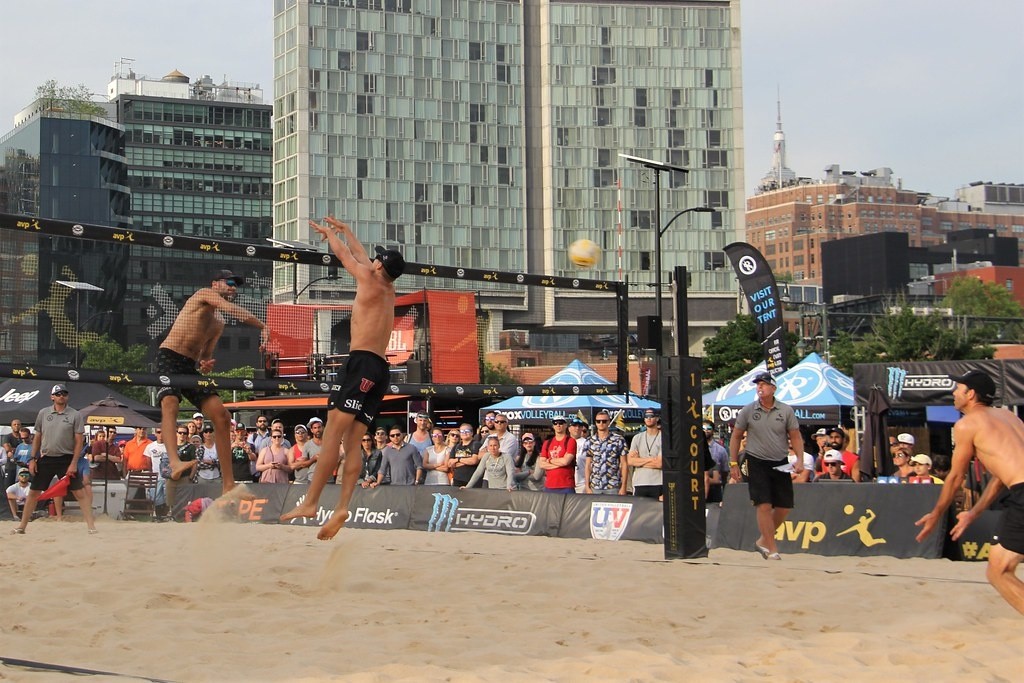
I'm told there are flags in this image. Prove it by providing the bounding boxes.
[722,242,788,377]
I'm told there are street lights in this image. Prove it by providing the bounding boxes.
[653,205,717,356]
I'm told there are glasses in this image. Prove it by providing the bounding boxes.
[824,462,839,467]
[432,416,507,439]
[889,441,899,447]
[702,425,713,430]
[523,438,533,442]
[552,414,655,433]
[756,374,775,383]
[892,453,904,458]
[52,391,69,397]
[416,414,429,419]
[224,279,239,288]
[18,420,304,477]
[362,431,401,441]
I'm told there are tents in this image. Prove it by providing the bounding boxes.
[479,359,661,437]
[854,359,1024,482]
[700,359,790,444]
[712,352,867,456]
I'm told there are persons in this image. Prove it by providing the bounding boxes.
[916,369,1024,613]
[280,213,405,540]
[728,373,803,562]
[153,270,269,502]
[0,408,979,521]
[9,383,99,535]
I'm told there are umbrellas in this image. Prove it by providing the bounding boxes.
[0,378,160,518]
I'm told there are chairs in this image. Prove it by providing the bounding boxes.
[124,472,158,521]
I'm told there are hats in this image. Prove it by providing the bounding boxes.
[50,384,69,394]
[192,413,324,432]
[811,428,846,466]
[643,407,659,416]
[374,246,405,279]
[213,269,244,286]
[752,372,777,386]
[910,453,932,466]
[948,370,996,403]
[897,433,914,444]
[18,468,29,475]
[554,416,567,423]
[522,433,534,442]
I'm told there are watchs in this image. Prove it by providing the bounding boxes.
[729,462,737,467]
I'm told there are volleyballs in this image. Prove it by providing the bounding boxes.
[568,239,601,267]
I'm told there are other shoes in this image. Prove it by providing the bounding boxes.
[753,542,782,562]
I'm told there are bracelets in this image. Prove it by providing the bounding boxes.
[458,458,461,462]
[549,458,552,464]
[27,457,37,465]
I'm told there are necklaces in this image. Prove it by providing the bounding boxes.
[645,431,659,452]
